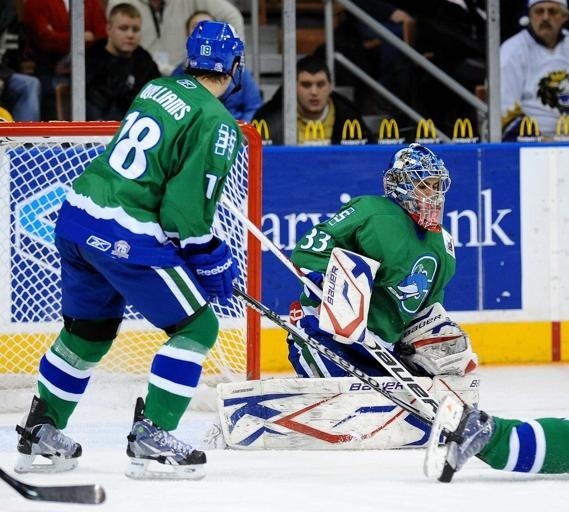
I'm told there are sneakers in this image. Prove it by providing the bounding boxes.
[439,404,496,482]
[17,396,81,460]
[128,398,206,466]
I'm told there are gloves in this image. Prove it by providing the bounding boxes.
[189,240,240,306]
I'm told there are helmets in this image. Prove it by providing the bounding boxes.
[383,143,451,233]
[187,20,245,75]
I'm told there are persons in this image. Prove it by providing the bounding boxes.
[252,56,376,144]
[285,143,456,377]
[314,1,569,141]
[16,21,244,482]
[1,0,261,123]
[423,395,568,482]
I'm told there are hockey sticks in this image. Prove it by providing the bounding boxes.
[1,468,106,505]
